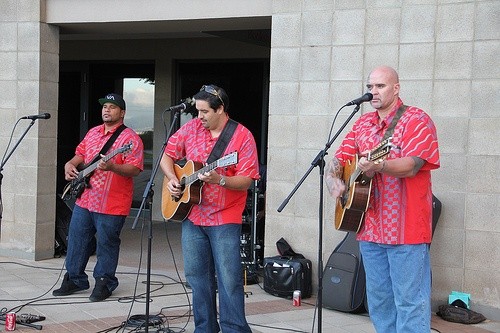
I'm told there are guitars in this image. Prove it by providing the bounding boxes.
[71,140,133,198]
[334,136,393,234]
[161,150,239,222]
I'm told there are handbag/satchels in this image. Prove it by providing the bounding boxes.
[436,299,487,324]
[263,255,313,299]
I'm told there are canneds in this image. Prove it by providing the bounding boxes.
[293,290,301,306]
[5,312,17,331]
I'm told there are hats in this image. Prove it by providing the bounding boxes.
[98,94,125,110]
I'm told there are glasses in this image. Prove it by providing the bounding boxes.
[200,85,225,106]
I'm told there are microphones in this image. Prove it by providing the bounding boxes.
[21,113,51,119]
[165,102,189,112]
[346,93,373,106]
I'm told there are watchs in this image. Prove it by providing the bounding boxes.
[218,174,226,186]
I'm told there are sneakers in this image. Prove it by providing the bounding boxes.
[90,277,119,302]
[53,277,89,296]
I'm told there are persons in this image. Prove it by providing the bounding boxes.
[52,94,143,302]
[159,85,259,333]
[325,66,440,333]
